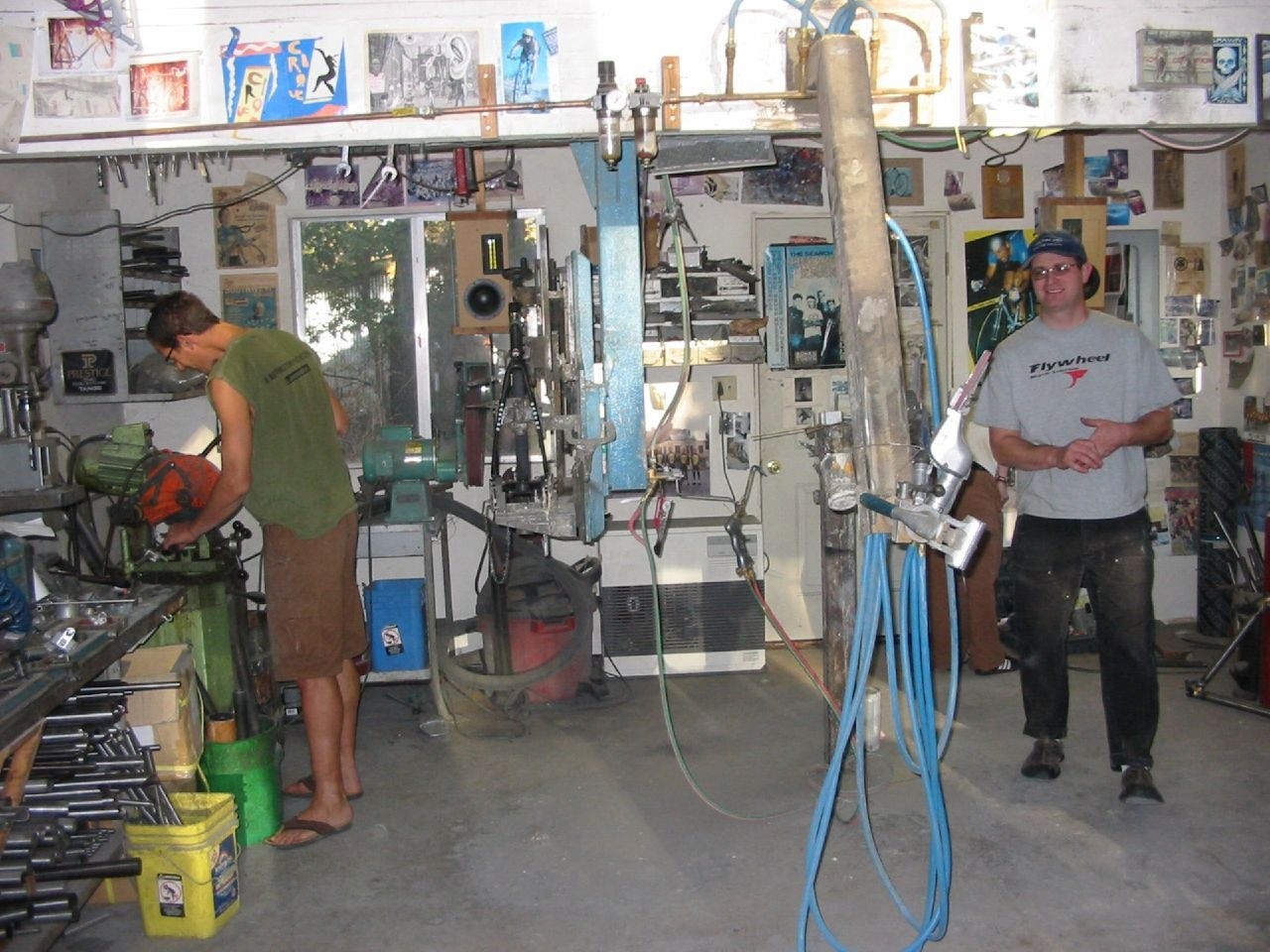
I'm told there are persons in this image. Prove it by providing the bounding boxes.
[1152,38,1202,89]
[655,445,704,489]
[145,291,369,850]
[789,290,845,364]
[508,28,539,85]
[971,231,1031,329]
[976,230,1183,804]
[247,301,273,328]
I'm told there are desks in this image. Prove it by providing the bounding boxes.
[355,507,455,715]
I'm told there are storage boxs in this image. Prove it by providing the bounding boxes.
[81,642,201,906]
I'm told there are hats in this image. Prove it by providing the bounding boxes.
[1026,230,1100,300]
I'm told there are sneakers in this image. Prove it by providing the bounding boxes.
[1119,767,1164,804]
[1020,738,1064,779]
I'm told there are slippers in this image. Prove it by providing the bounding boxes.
[266,815,351,850]
[283,774,364,801]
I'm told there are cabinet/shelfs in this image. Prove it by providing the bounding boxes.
[41,208,205,403]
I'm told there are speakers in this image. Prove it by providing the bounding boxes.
[446,211,516,336]
[1038,197,1106,310]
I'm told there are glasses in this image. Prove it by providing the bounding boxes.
[164,343,176,366]
[1032,263,1079,281]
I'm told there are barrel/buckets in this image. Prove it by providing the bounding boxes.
[478,606,593,704]
[124,792,241,939]
[363,577,429,673]
[200,714,285,846]
